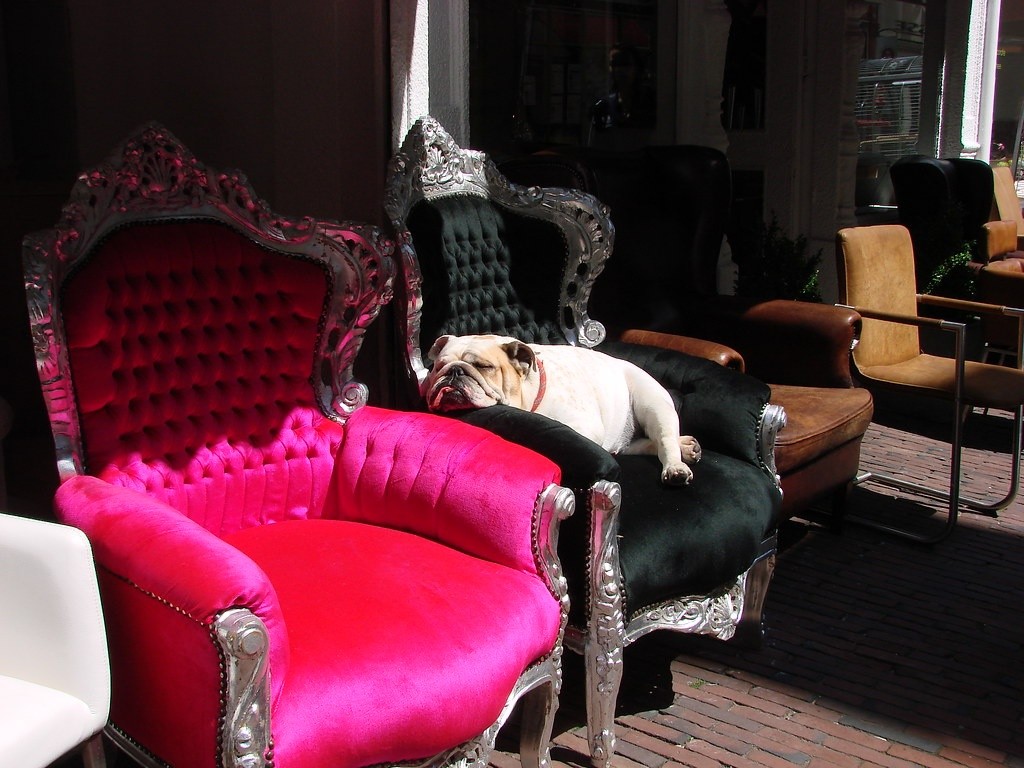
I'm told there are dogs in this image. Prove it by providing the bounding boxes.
[427,335,702,486]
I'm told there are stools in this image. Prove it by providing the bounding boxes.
[1,512,111,768]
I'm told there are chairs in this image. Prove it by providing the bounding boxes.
[20,121,575,768]
[834,155,1024,546]
[383,114,787,768]
[524,144,876,526]
